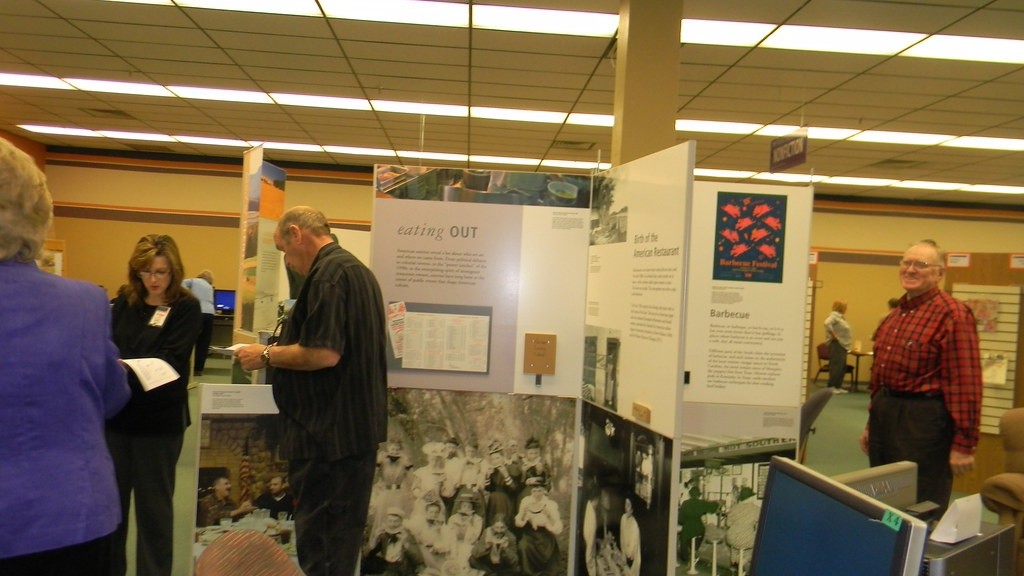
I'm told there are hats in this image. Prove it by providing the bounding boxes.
[387,507,405,518]
[526,477,548,487]
[443,437,459,445]
[423,442,450,459]
[487,436,504,454]
[454,494,480,504]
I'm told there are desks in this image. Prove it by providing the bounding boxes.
[850,351,874,392]
[212,315,234,326]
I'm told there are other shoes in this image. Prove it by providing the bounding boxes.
[830,386,848,394]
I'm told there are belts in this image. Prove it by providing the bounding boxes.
[879,385,943,398]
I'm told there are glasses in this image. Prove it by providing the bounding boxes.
[901,259,941,269]
[137,268,171,279]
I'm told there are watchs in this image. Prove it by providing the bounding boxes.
[260,345,274,368]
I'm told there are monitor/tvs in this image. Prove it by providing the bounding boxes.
[214,289,235,315]
[747,455,927,576]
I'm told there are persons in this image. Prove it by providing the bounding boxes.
[858,241,982,521]
[200,476,295,528]
[233,206,389,576]
[636,444,653,510]
[181,271,215,377]
[362,435,580,564]
[678,477,760,570]
[582,478,641,576]
[824,298,899,394]
[0,135,132,576]
[104,235,201,576]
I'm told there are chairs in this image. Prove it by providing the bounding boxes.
[194,528,308,576]
[814,343,854,386]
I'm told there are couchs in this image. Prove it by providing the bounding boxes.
[981,407,1023,576]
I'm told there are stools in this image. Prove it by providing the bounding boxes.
[734,546,750,576]
[707,538,723,576]
[686,536,701,576]
